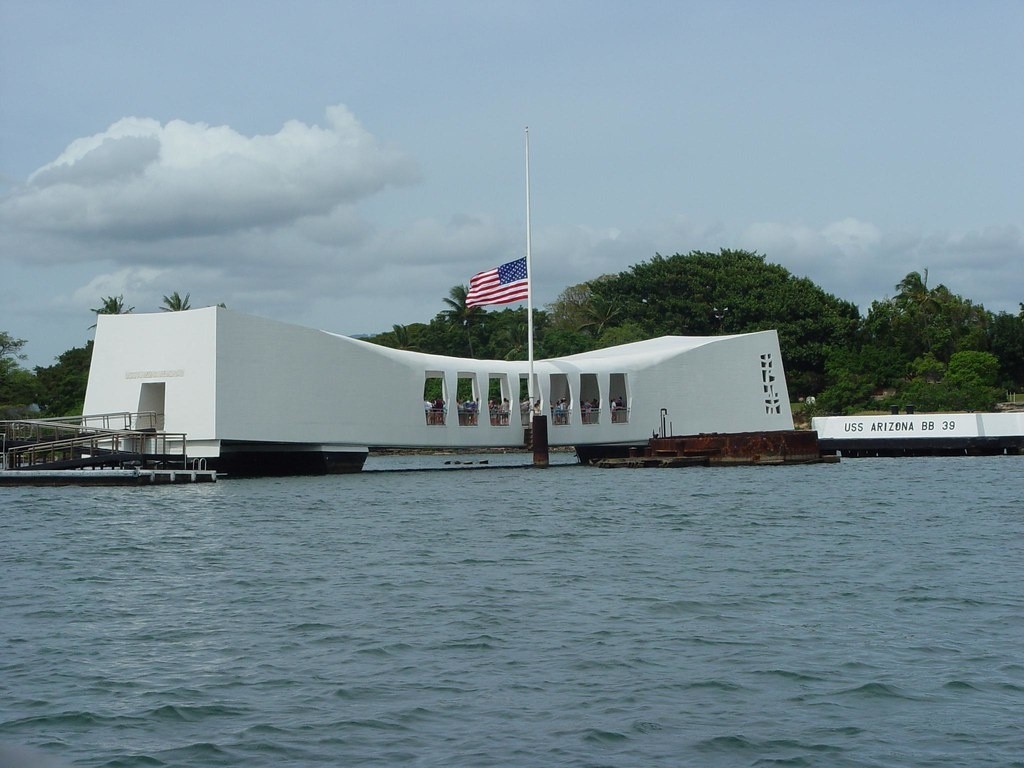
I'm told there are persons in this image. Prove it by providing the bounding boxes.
[457,398,479,426]
[489,397,509,426]
[580,399,599,424]
[550,397,570,425]
[424,398,447,425]
[520,393,540,426]
[610,396,622,421]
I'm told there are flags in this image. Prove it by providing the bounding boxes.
[465,255,528,309]
